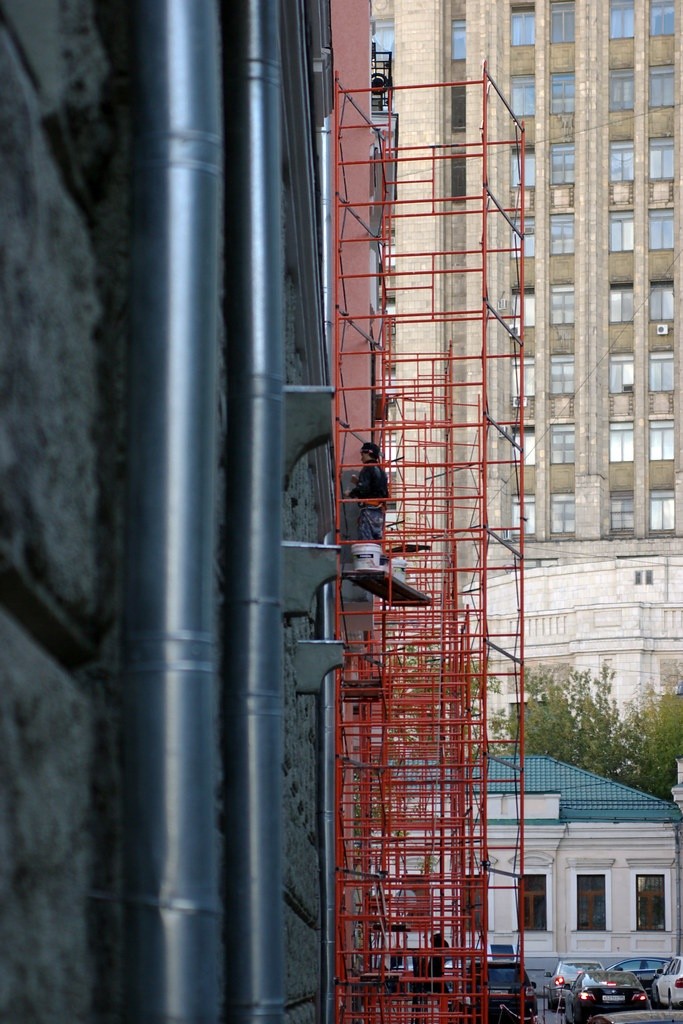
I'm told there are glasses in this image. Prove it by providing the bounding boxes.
[360,448,373,454]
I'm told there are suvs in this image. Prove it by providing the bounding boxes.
[392,956,538,1024]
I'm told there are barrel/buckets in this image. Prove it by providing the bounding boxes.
[351,543,381,570]
[391,558,407,583]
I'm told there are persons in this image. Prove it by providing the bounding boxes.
[427,933,450,994]
[343,442,389,543]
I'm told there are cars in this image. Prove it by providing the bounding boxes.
[544,960,605,1013]
[587,956,683,1024]
[563,971,652,1024]
